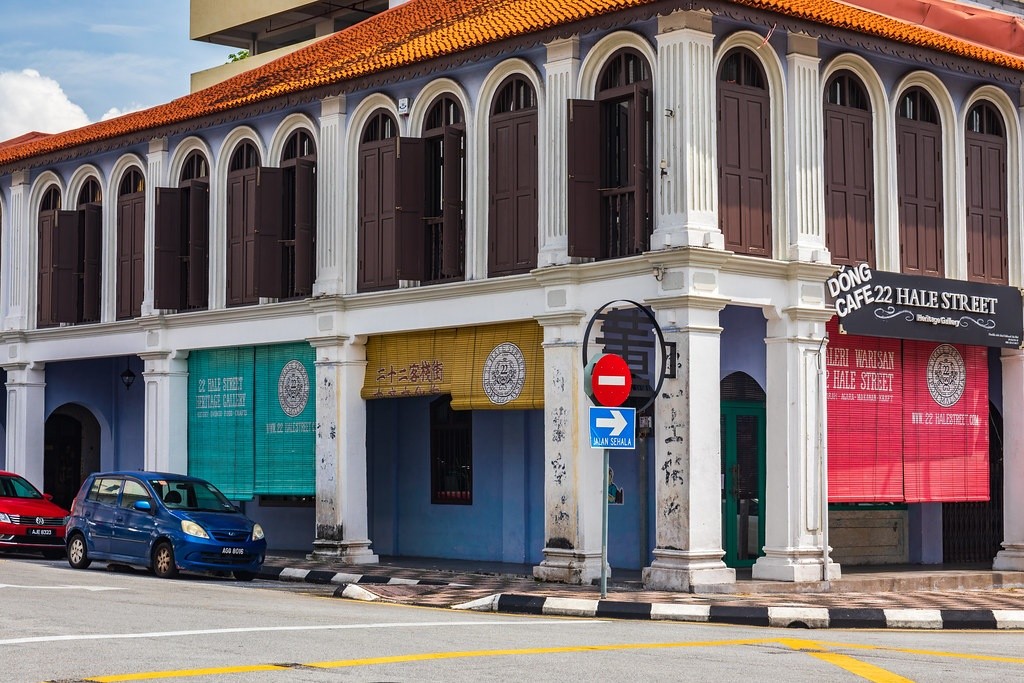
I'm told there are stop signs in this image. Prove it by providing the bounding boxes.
[591,354,632,406]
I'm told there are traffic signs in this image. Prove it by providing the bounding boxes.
[588,407,636,451]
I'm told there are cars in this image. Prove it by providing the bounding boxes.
[63,471,268,583]
[0,470,74,559]
